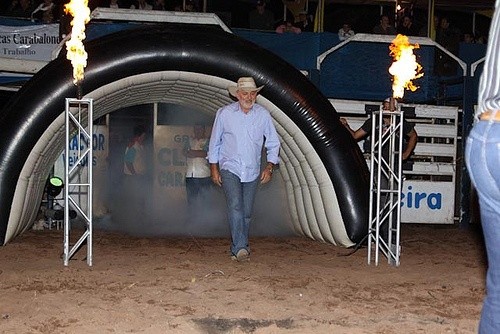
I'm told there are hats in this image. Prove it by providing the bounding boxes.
[382,97,398,108]
[229,77,264,97]
[257,0,264,6]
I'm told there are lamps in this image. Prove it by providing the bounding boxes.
[46,177,64,197]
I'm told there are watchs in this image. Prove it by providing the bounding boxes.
[265,166,274,173]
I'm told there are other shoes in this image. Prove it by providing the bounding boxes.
[231,248,250,262]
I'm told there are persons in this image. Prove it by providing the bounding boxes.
[340,96,419,258]
[181,122,211,224]
[465,0,500,334]
[122,125,154,212]
[206,77,280,262]
